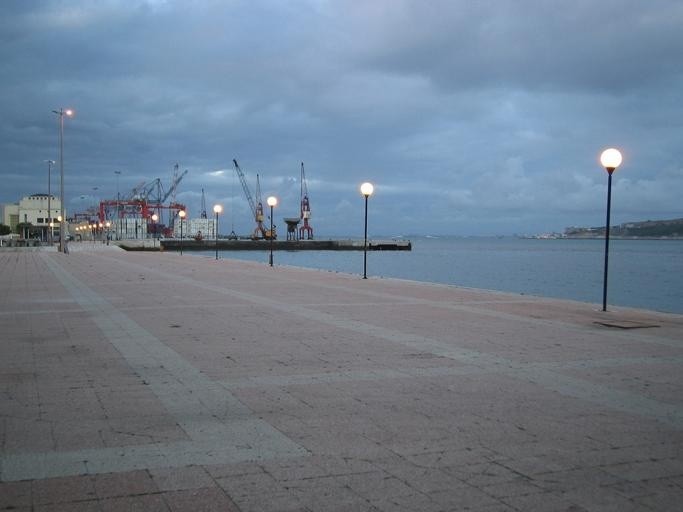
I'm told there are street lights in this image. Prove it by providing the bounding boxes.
[150,214,158,250]
[359,180,376,280]
[79,195,90,209]
[213,203,223,260]
[178,210,186,255]
[75,222,111,241]
[266,195,278,267]
[51,105,75,252]
[44,160,56,246]
[598,148,623,312]
[113,169,122,241]
[92,184,103,192]
